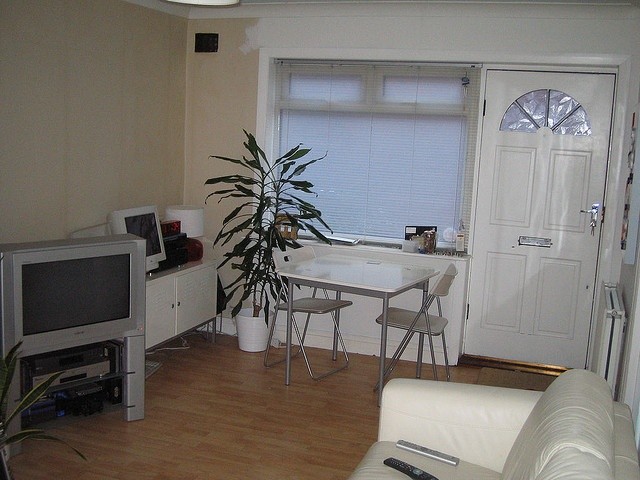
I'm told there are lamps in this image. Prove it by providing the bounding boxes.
[166,205,204,238]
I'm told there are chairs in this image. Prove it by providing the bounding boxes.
[262,246,350,380]
[374,263,459,392]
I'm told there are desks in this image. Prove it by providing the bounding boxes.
[279,254,440,408]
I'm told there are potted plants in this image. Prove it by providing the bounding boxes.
[201,129,333,353]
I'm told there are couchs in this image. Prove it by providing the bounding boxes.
[347,369,639,480]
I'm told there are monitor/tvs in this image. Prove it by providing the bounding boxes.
[106,205,167,273]
[0,232,149,361]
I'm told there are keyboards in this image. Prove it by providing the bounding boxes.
[145,359,163,379]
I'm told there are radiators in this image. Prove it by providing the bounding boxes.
[591,282,628,402]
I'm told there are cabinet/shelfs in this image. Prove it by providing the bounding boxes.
[145,260,218,352]
[1,336,146,458]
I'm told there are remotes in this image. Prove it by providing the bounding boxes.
[383,456,440,480]
[396,439,460,466]
